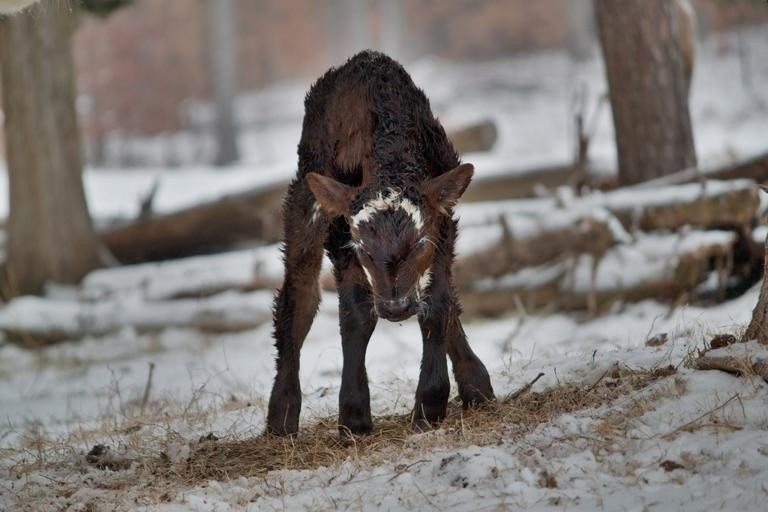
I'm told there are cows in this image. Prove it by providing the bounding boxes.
[267,47,497,448]
